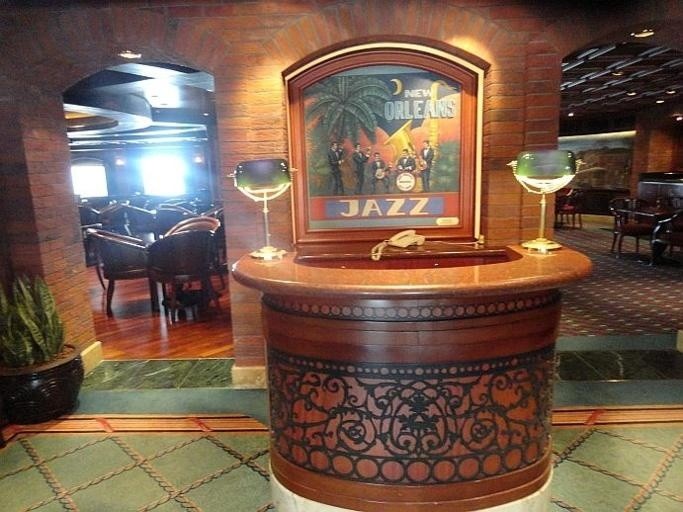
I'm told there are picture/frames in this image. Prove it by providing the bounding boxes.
[286,49,477,244]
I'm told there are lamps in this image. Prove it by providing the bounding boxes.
[504,150,585,251]
[225,158,297,260]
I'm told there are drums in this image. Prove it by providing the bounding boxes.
[396,170,415,191]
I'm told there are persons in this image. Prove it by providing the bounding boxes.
[368,152,392,194]
[327,141,345,195]
[351,143,371,194]
[419,140,435,192]
[395,148,416,173]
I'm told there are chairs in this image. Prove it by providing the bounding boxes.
[554,171,682,269]
[77,188,230,325]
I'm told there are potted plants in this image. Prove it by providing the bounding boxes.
[0,268,83,427]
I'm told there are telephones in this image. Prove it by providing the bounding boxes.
[387,229,425,248]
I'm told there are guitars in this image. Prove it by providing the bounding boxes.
[409,142,427,171]
